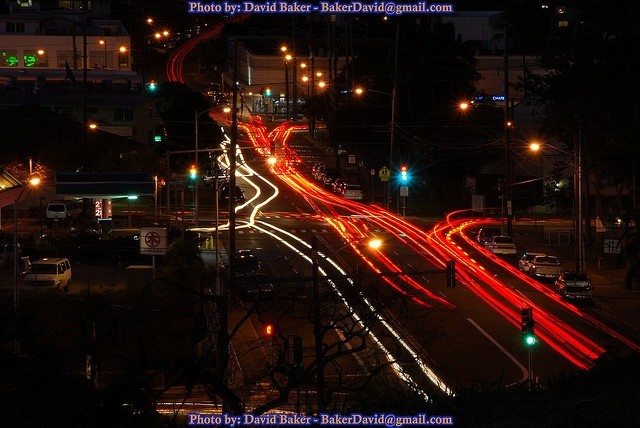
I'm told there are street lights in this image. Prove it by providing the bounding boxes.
[527,130,583,277]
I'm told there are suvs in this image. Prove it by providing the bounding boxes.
[477,228,500,243]
[344,184,364,200]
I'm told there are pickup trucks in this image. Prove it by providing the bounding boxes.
[530,254,562,279]
[555,272,593,302]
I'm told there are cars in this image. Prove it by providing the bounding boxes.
[312,161,341,193]
[221,185,245,204]
[230,250,261,279]
[484,236,516,255]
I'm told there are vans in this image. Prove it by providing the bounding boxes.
[0,244,16,265]
[24,257,72,293]
[518,252,545,272]
[46,200,74,222]
